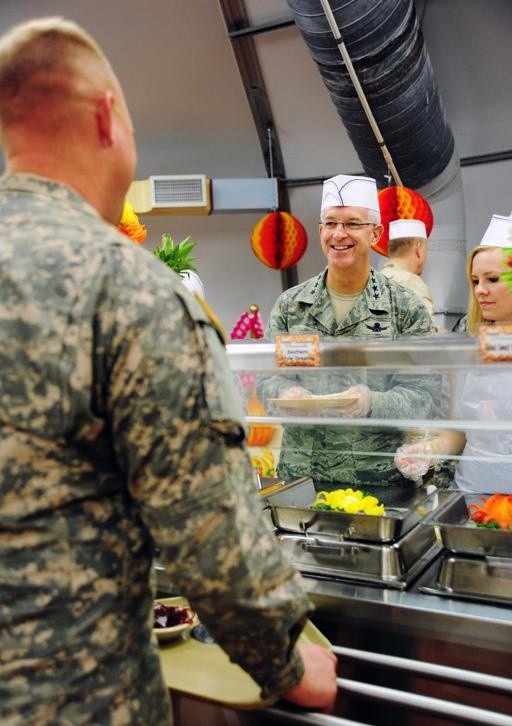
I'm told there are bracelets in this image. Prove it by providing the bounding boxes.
[428,441,440,470]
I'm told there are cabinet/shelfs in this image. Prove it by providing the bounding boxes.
[226,334,511,726]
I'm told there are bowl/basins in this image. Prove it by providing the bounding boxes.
[152,597,198,647]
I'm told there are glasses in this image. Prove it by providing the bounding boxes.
[322,220,376,229]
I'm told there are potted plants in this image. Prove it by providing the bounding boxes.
[154,232,205,304]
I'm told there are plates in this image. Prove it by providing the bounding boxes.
[267,394,359,410]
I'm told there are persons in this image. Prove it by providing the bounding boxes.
[255,173,451,486]
[0,15,338,726]
[394,214,512,495]
[379,218,434,325]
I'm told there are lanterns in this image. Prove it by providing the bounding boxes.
[370,185,433,258]
[250,211,308,271]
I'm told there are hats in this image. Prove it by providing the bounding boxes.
[321,175,380,211]
[389,218,427,240]
[480,214,512,247]
